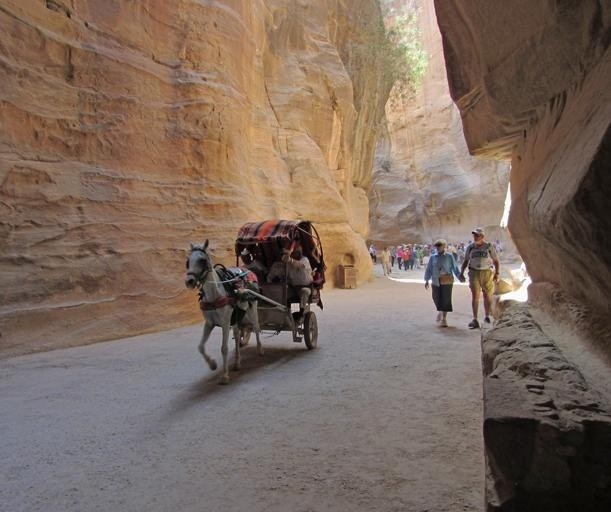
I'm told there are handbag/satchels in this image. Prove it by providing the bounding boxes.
[438,275,453,285]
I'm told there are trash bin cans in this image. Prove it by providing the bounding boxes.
[338,264,359,288]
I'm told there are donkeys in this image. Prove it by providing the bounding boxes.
[185,238,266,385]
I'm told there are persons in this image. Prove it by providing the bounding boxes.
[239,241,313,324]
[424,238,465,327]
[457,228,500,327]
[370,240,501,279]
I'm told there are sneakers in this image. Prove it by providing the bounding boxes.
[468,319,479,327]
[439,320,448,327]
[483,317,490,324]
[436,312,441,321]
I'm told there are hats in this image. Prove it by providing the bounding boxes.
[435,239,447,247]
[471,228,485,236]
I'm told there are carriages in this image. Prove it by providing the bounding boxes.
[184,213,327,389]
